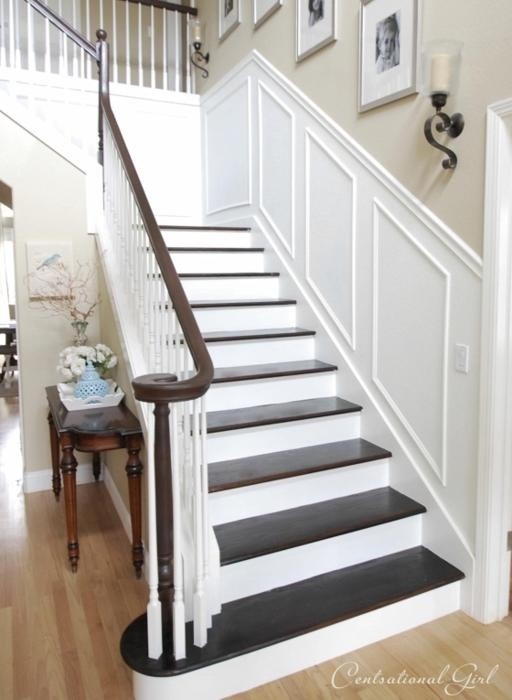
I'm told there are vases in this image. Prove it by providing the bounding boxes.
[74,364,109,396]
[71,323,90,348]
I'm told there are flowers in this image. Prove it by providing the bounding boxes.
[59,343,118,377]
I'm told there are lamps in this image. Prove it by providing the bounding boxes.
[190,22,210,79]
[420,53,465,170]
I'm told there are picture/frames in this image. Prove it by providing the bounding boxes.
[357,0,421,115]
[218,0,241,42]
[253,0,284,30]
[296,0,338,64]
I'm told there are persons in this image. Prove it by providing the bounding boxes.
[308,0,323,27]
[376,16,398,75]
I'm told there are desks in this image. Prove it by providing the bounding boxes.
[45,385,145,580]
[0,324,18,388]
